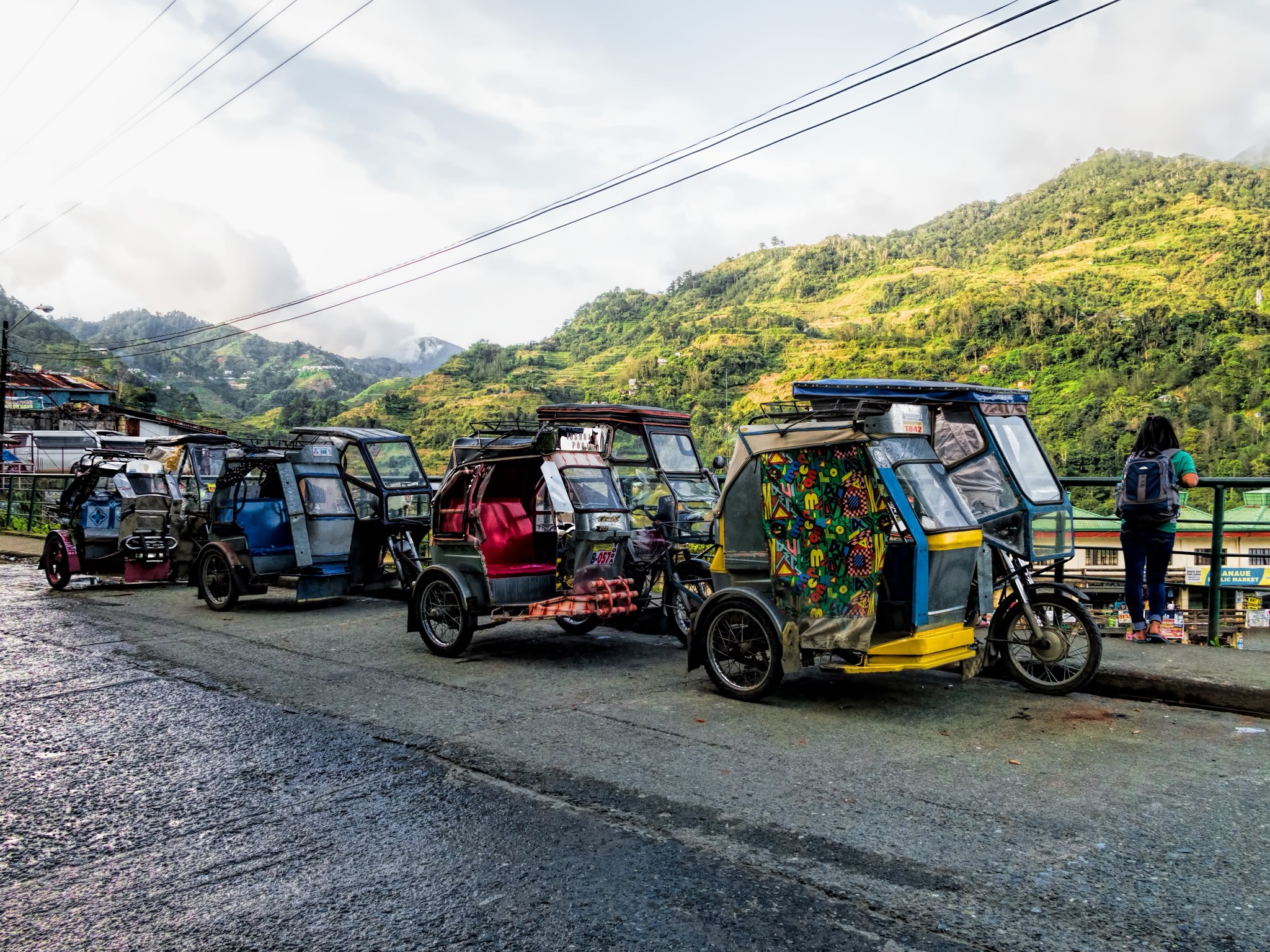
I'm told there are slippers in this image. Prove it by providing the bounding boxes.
[1132,633,1148,643]
[1149,634,1170,643]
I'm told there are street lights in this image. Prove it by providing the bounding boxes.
[0,305,54,492]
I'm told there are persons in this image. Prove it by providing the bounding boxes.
[1114,416,1198,643]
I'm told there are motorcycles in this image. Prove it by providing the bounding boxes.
[188,426,432,613]
[406,403,726,658]
[684,378,1102,704]
[37,433,244,591]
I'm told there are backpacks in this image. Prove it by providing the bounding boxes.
[1118,447,1180,523]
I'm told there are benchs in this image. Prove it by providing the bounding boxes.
[221,499,295,570]
[444,496,558,579]
[80,497,122,544]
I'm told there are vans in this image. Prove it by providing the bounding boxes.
[3,430,128,479]
